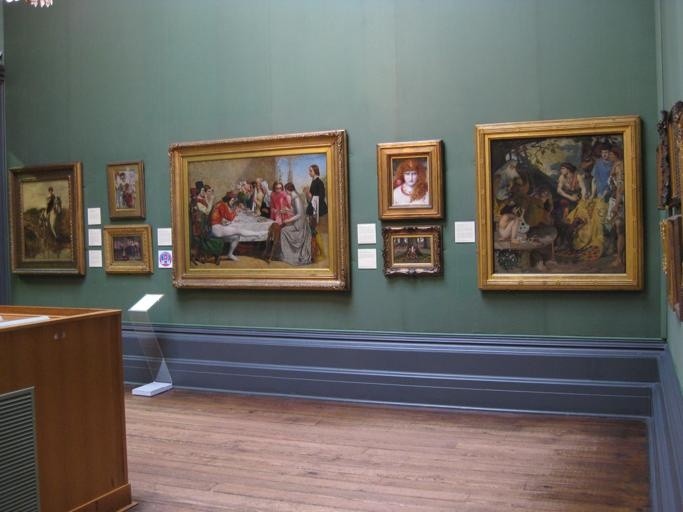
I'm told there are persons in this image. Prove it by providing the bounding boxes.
[190,164,327,265]
[498,142,624,272]
[118,172,135,208]
[47,186,56,213]
[114,238,141,259]
[394,158,429,204]
[38,207,47,232]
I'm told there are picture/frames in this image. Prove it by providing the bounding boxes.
[473,115,648,291]
[100,160,155,276]
[6,161,85,276]
[169,128,351,293]
[375,139,447,281]
